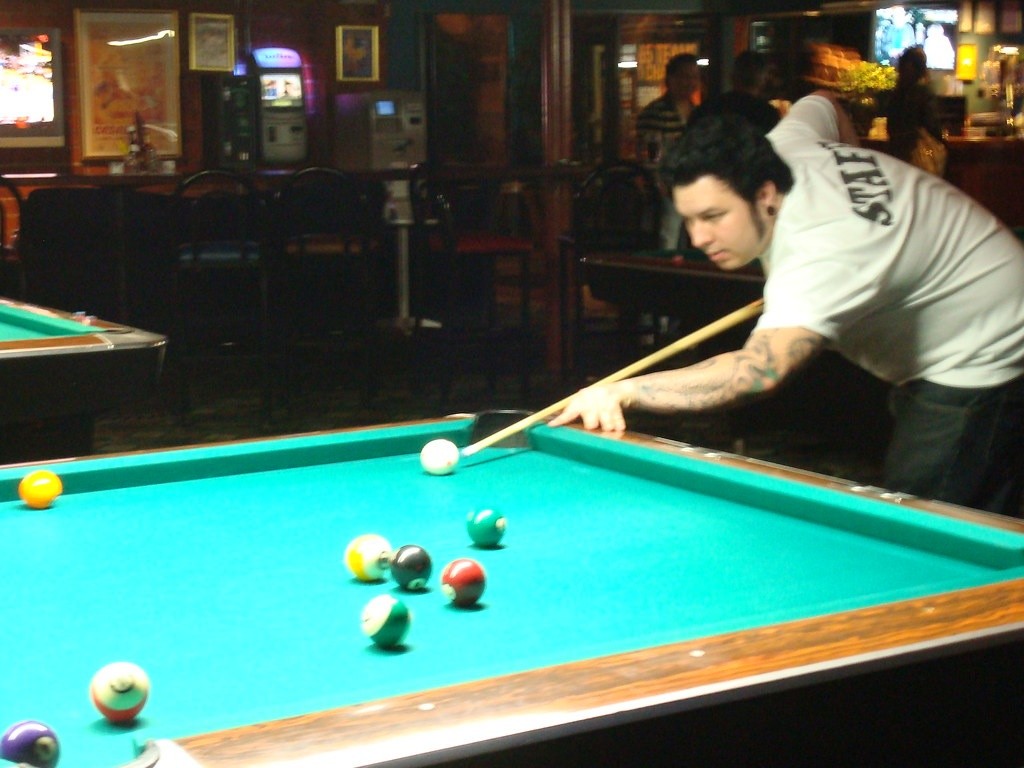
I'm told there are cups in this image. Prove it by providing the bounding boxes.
[160,159,177,175]
[109,161,125,175]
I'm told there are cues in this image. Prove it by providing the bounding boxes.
[462,297,766,459]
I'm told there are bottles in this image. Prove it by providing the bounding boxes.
[127,122,157,173]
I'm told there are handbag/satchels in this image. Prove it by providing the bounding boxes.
[911,127,946,177]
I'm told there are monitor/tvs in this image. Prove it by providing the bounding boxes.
[0,27,65,148]
[868,6,959,75]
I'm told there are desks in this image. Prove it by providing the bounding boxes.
[0,410,1024,768]
[0,293,170,464]
[573,240,763,457]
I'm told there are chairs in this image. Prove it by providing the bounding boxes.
[172,159,664,424]
[0,176,32,302]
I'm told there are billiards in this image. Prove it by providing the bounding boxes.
[466,509,505,548]
[0,721,59,768]
[361,596,411,647]
[345,532,486,602]
[420,438,460,476]
[90,661,151,725]
[17,469,63,511]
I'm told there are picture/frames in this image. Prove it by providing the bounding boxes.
[74,7,184,162]
[188,12,236,72]
[335,25,379,81]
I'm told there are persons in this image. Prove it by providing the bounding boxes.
[887,47,948,177]
[663,39,860,256]
[636,54,703,347]
[549,90,1024,504]
